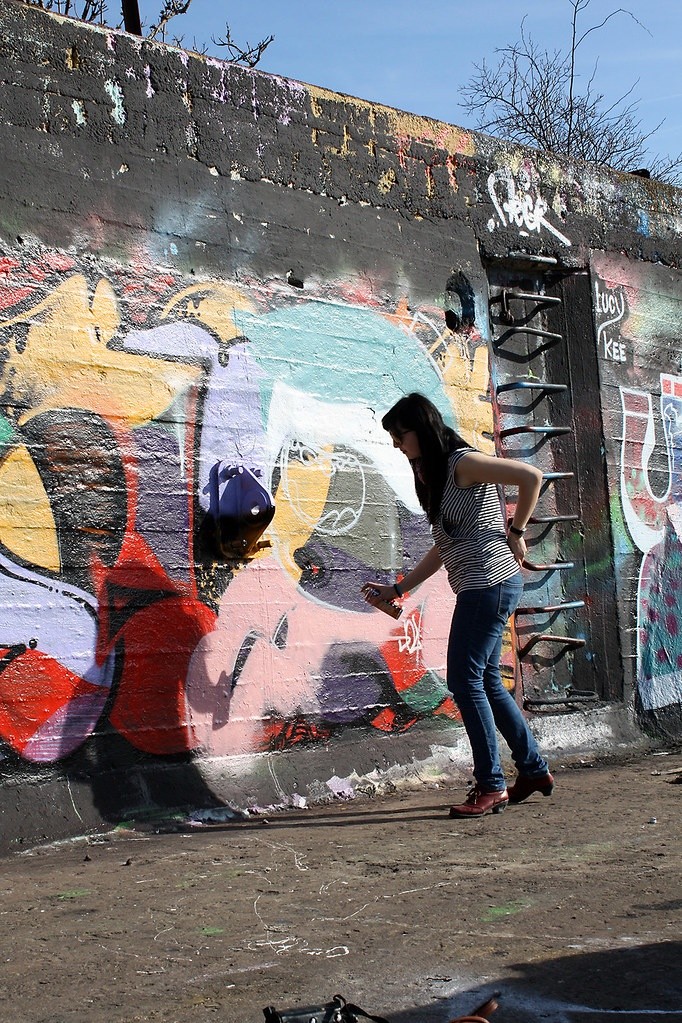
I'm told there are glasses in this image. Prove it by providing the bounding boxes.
[392,428,412,446]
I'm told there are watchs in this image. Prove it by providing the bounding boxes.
[509,525,526,537]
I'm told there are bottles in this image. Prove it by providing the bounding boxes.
[362,588,403,620]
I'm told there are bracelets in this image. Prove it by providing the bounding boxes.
[393,584,402,599]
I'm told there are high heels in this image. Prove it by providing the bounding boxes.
[507,770,554,806]
[450,785,509,817]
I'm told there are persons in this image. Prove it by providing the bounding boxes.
[360,393,560,818]
